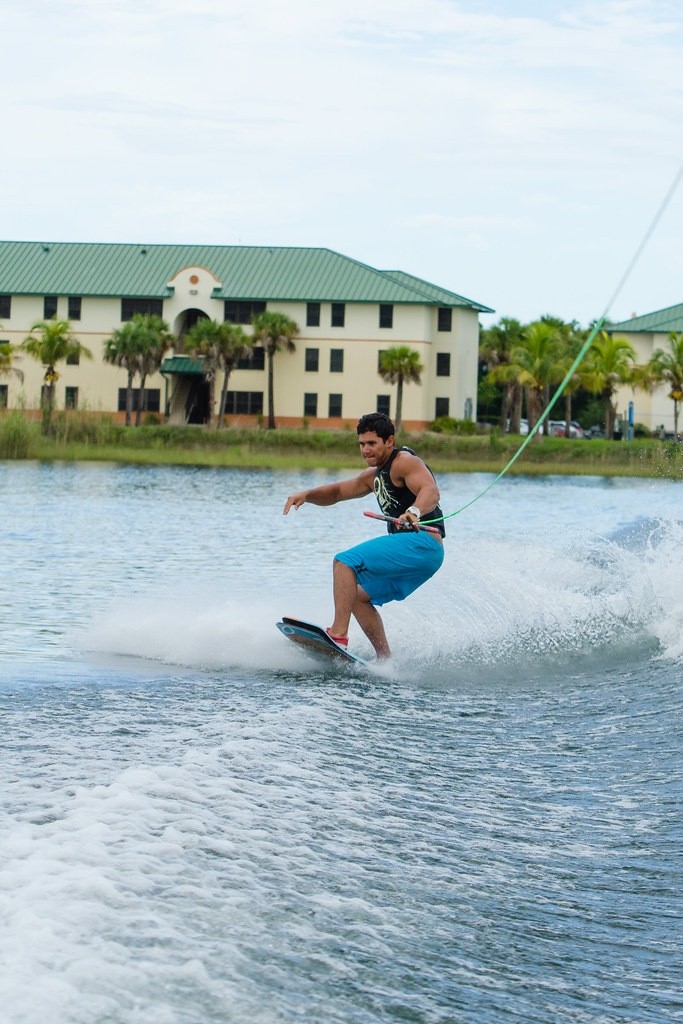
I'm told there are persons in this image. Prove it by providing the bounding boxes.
[283,414,446,667]
[658,425,665,441]
[626,423,635,440]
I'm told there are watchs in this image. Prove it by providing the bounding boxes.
[405,505,420,520]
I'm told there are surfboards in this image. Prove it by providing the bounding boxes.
[276,617,371,665]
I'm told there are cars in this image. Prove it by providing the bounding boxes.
[506,419,601,440]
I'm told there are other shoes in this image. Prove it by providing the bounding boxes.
[324,627,349,651]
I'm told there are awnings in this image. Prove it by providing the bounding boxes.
[159,357,216,381]
